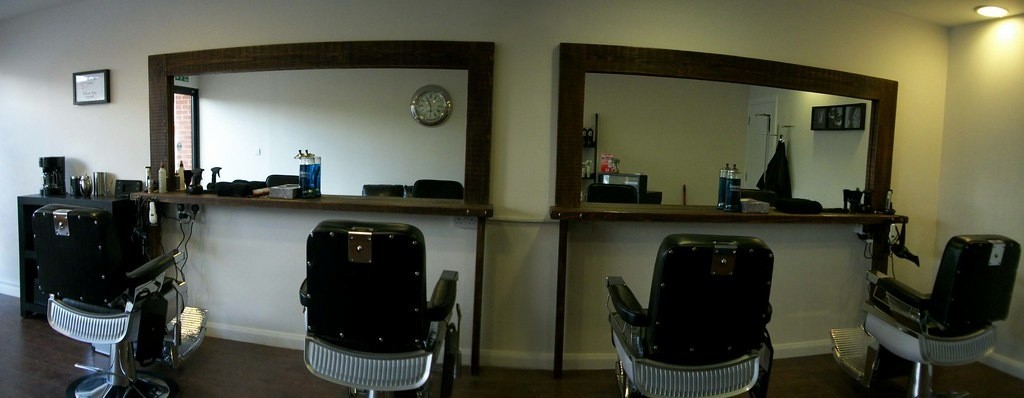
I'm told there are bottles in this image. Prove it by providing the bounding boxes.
[178,160,185,190]
[582,124,593,146]
[724,163,741,211]
[70,173,92,197]
[158,162,167,193]
[93,171,106,197]
[718,162,733,208]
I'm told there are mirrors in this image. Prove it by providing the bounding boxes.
[556,41,900,211]
[147,41,495,202]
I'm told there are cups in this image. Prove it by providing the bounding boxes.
[305,150,322,199]
[294,149,305,197]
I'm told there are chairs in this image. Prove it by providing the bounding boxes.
[32,202,209,398]
[412,179,465,199]
[829,234,1022,398]
[586,184,639,204]
[607,234,774,398]
[362,184,405,198]
[299,219,462,398]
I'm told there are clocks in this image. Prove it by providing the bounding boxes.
[410,84,455,125]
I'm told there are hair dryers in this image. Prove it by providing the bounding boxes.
[891,223,920,268]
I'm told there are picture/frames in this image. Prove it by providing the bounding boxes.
[811,102,867,131]
[72,69,111,105]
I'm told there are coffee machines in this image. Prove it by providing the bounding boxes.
[38,156,66,196]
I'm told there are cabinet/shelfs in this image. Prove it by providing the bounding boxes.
[17,193,145,320]
[581,114,599,182]
